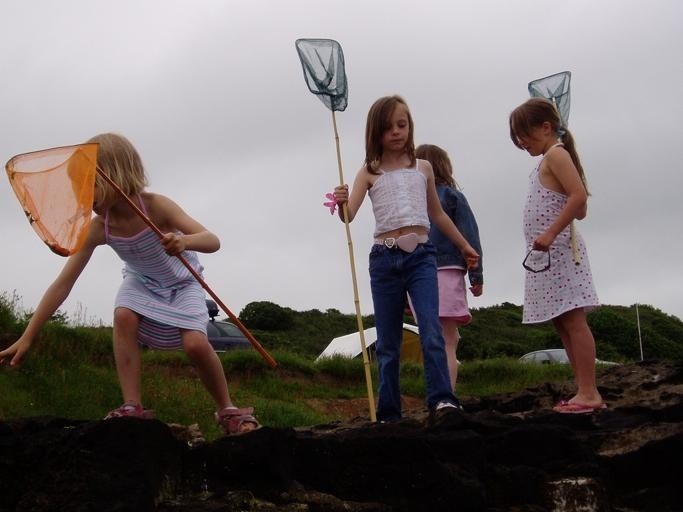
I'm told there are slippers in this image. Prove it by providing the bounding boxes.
[553,399,607,414]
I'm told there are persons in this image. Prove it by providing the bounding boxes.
[509,98,603,413]
[415,145,483,395]
[335,95,480,422]
[0,131,262,432]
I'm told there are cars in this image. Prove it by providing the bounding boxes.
[204,299,256,353]
[518,349,620,366]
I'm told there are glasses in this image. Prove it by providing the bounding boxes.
[522,250,551,272]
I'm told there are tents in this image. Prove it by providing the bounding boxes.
[313,320,464,363]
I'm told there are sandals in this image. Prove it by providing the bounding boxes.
[212,408,261,436]
[104,401,151,419]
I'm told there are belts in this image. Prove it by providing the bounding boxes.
[373,234,429,249]
